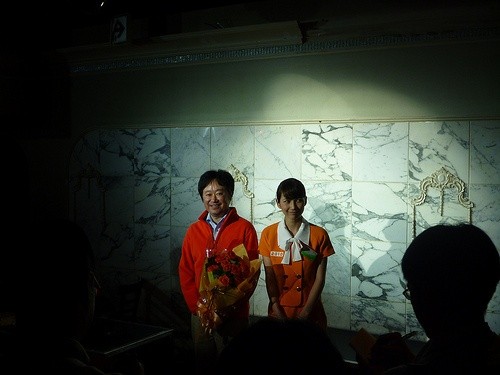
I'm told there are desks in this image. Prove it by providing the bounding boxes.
[80,315,176,375]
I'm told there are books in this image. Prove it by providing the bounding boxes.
[349,327,384,375]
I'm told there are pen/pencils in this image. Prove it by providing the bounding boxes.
[381,331,417,354]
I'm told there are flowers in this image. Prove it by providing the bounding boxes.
[196,243,263,336]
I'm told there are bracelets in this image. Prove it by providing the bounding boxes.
[270,300,277,307]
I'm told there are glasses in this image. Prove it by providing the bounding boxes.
[403,287,427,302]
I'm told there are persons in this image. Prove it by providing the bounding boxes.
[259,179,334,332]
[218,317,345,375]
[180,169,260,375]
[356,225,500,375]
[0,219,103,375]
